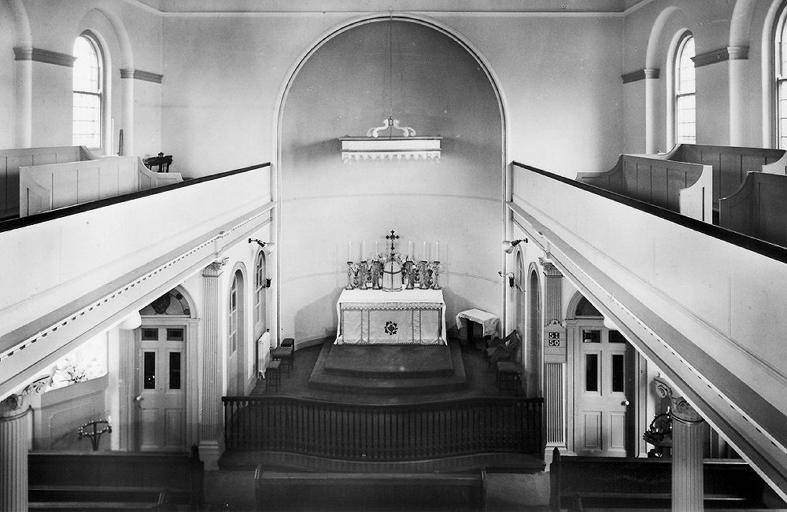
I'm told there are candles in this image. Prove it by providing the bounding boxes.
[348,239,440,264]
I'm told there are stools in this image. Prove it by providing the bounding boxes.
[266,338,294,392]
[496,360,519,387]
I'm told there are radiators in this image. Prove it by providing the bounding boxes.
[257,331,273,380]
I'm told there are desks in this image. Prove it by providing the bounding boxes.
[459,308,499,353]
[336,286,446,346]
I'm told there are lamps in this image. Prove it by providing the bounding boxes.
[338,7,443,164]
[248,238,272,258]
[502,238,527,254]
